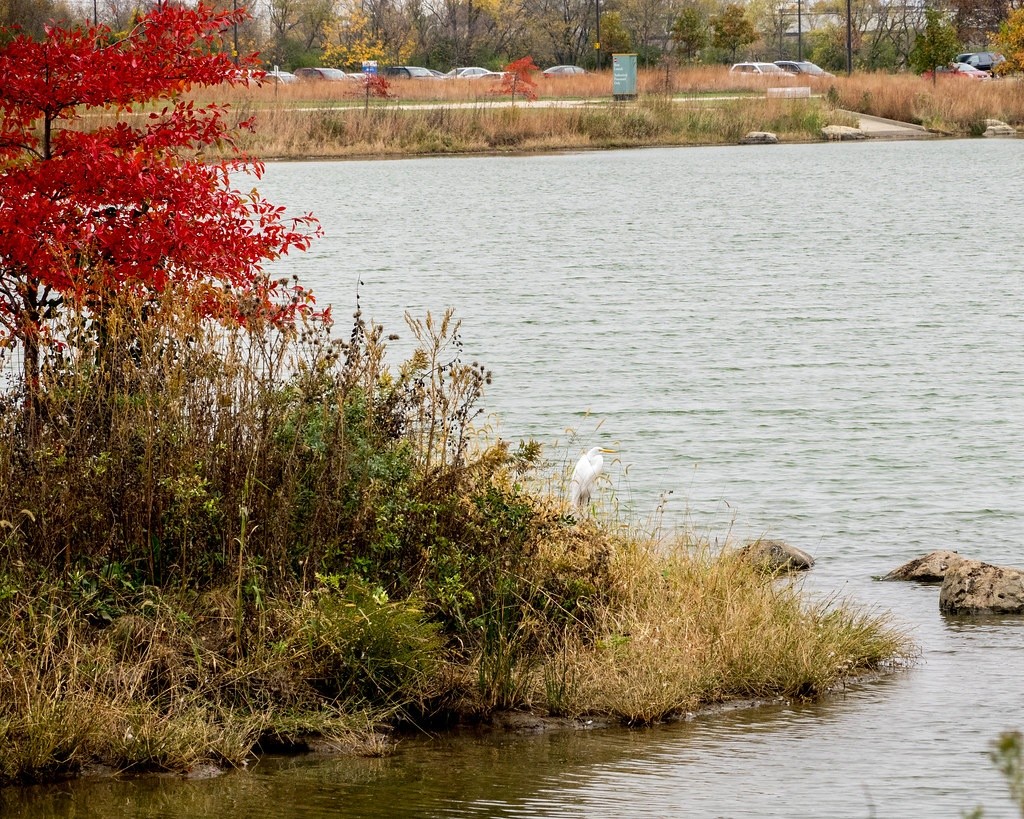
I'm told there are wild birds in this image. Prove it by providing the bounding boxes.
[567,446,621,521]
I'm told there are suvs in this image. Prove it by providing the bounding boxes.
[293,68,349,81]
[953,51,1006,78]
[385,66,438,79]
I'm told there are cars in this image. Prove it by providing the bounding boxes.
[773,60,835,78]
[729,62,795,78]
[224,68,300,86]
[542,65,589,78]
[478,71,511,79]
[446,67,492,79]
[922,61,991,81]
[347,73,375,82]
[429,70,450,80]
